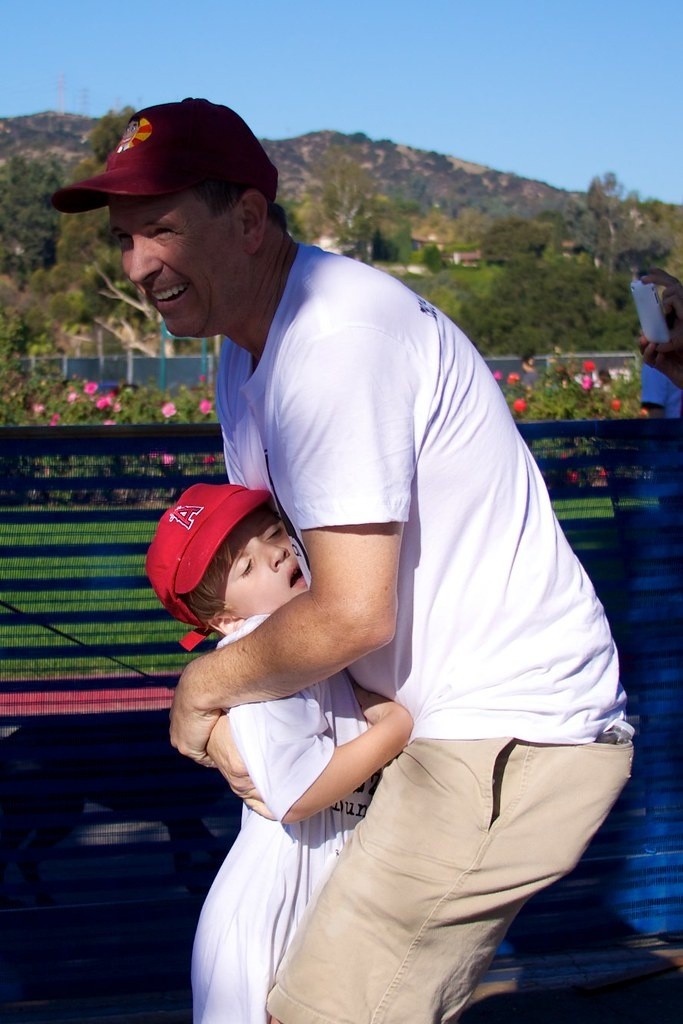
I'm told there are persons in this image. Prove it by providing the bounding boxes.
[636,267,683,422]
[141,482,415,1024]
[519,346,617,394]
[98,93,636,1024]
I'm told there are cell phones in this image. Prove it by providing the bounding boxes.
[629,280,672,345]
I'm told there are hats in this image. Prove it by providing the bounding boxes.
[52,98,279,214]
[145,483,272,651]
